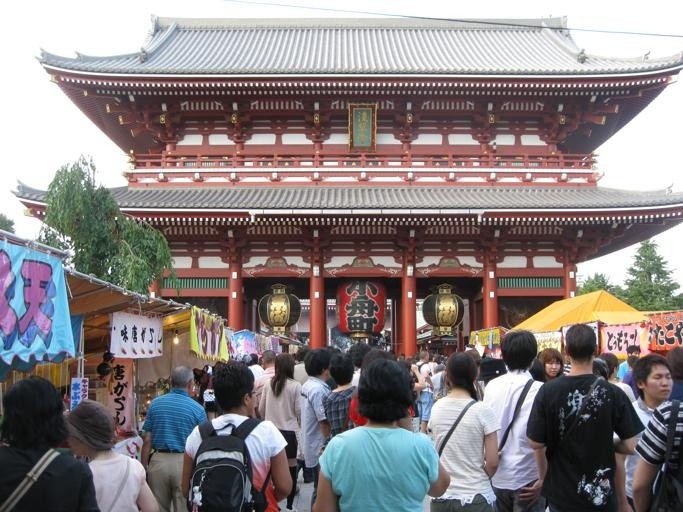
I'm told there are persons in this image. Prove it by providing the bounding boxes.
[63,398,161,512]
[0,374,99,511]
[139,323,683,512]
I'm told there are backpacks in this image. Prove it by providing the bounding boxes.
[187,419,270,512]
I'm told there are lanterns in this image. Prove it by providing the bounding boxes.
[256,284,302,339]
[420,284,471,336]
[336,280,389,342]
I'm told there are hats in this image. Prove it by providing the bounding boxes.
[65,399,113,449]
[478,358,507,382]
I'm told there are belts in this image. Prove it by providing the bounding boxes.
[159,449,178,453]
